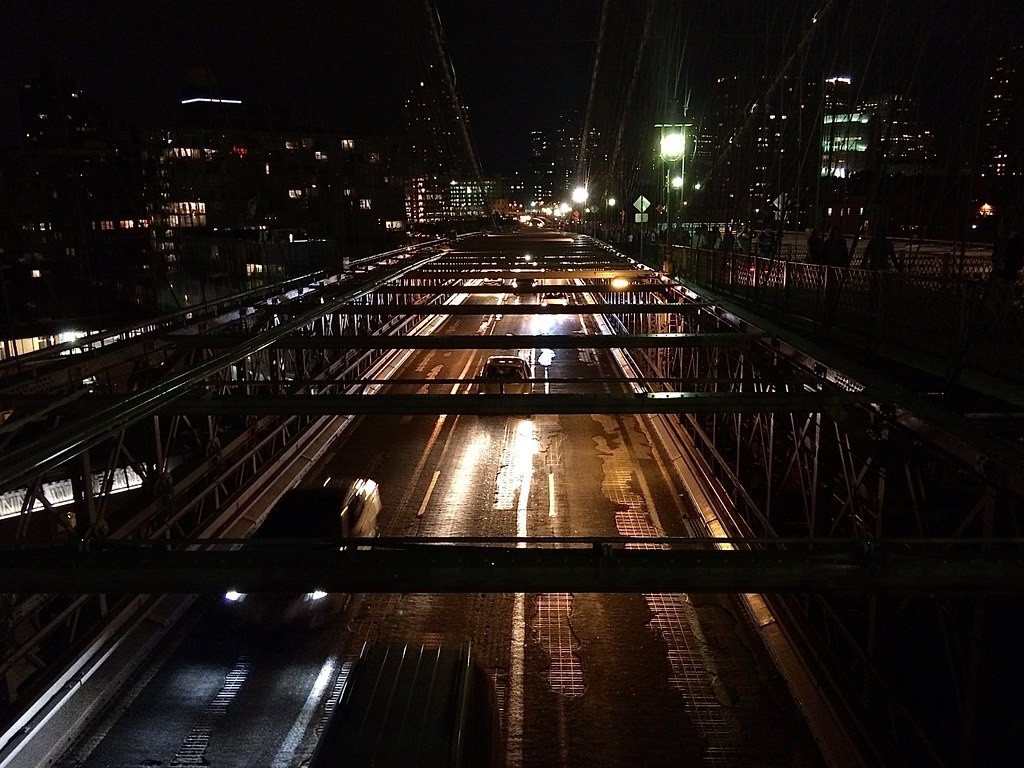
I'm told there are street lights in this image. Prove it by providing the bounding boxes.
[653,125,693,276]
[571,180,598,238]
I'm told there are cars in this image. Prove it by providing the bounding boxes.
[512,278,539,289]
[528,216,552,228]
[221,476,382,609]
[478,355,534,395]
[483,270,505,286]
[540,292,571,307]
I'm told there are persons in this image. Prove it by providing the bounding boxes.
[989,210,1024,337]
[865,227,903,318]
[804,225,848,301]
[594,221,776,269]
